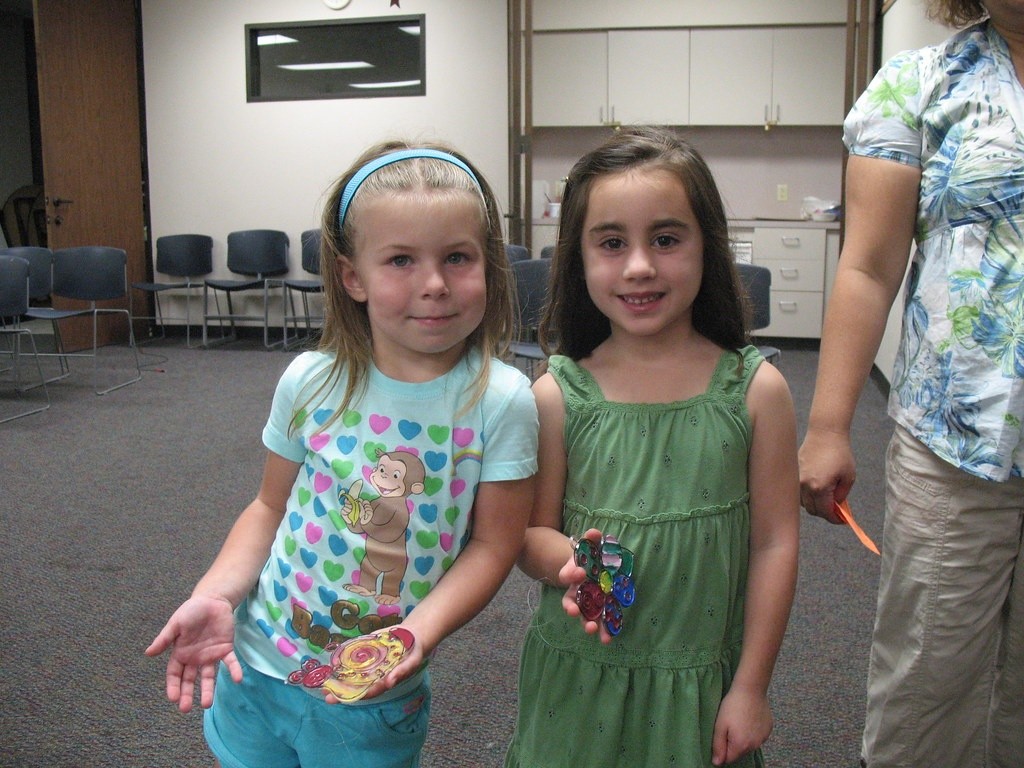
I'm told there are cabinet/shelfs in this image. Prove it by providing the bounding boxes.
[749,227,828,338]
[690,27,846,128]
[529,26,690,128]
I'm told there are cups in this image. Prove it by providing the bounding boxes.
[548,203,562,218]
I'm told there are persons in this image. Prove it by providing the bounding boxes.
[504,131,800,768]
[145,140,539,768]
[796,0,1024,768]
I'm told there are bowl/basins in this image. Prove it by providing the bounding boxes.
[805,201,840,221]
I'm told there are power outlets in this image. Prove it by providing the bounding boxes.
[777,183,788,201]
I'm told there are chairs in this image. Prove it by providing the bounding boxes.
[0,247,72,391]
[26,245,142,395]
[0,257,51,422]
[282,230,325,349]
[206,230,297,348]
[127,234,224,349]
[501,239,773,383]
